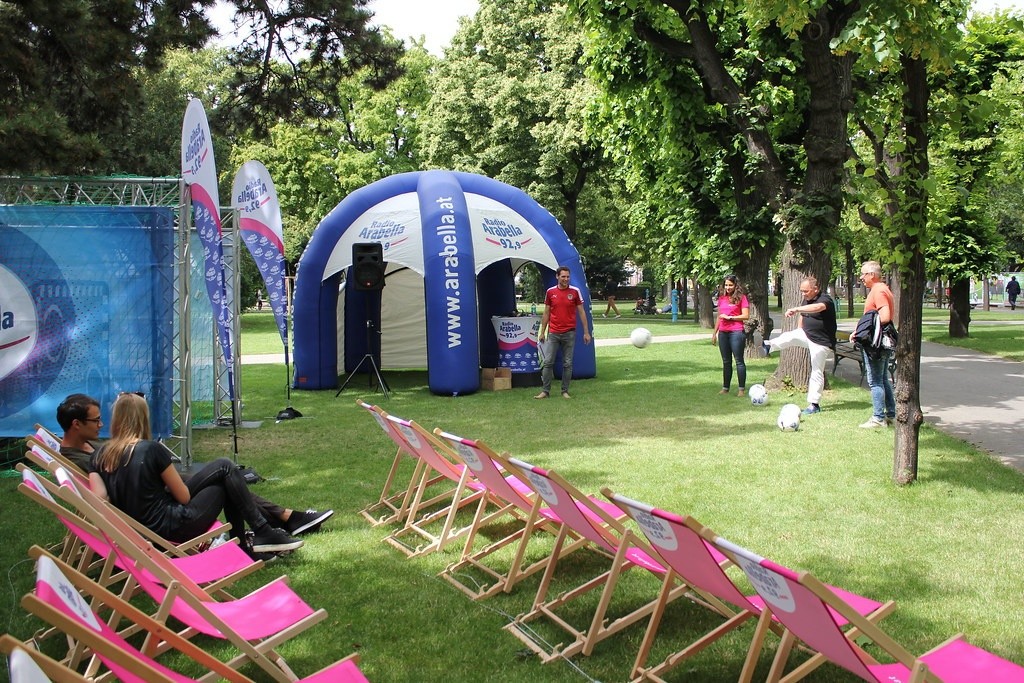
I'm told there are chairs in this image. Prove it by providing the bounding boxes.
[0,422,373,683]
[355,399,1024,683]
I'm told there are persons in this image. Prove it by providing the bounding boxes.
[1005,276,1021,310]
[597,274,622,318]
[534,267,591,398]
[86,392,304,565]
[56,393,333,538]
[754,277,837,414]
[849,261,897,428]
[712,275,750,396]
[636,297,656,317]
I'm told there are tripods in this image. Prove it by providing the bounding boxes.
[334,290,390,397]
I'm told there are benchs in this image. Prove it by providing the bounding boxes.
[925,294,951,310]
[833,340,897,388]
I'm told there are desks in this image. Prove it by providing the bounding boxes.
[492,314,545,387]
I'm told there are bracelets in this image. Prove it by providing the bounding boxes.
[729,316,733,320]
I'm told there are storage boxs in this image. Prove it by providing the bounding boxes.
[481,367,511,391]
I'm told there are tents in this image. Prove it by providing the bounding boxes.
[291,170,597,398]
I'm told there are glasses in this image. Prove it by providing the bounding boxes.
[117,391,146,401]
[86,416,101,423]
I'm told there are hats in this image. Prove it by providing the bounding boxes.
[607,275,612,279]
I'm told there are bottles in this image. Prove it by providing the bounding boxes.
[531,302,536,315]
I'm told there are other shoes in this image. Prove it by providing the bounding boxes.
[754,332,769,358]
[563,393,571,399]
[738,391,744,396]
[805,404,820,414]
[719,390,729,394]
[602,314,606,318]
[615,315,620,318]
[534,391,549,398]
[858,415,896,429]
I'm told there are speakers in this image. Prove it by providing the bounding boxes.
[353,243,385,290]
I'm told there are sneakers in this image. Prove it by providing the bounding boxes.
[248,550,276,564]
[253,527,304,553]
[289,509,334,537]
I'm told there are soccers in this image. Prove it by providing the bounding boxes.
[748,384,767,397]
[751,390,768,406]
[778,412,800,432]
[631,328,653,348]
[781,404,801,419]
